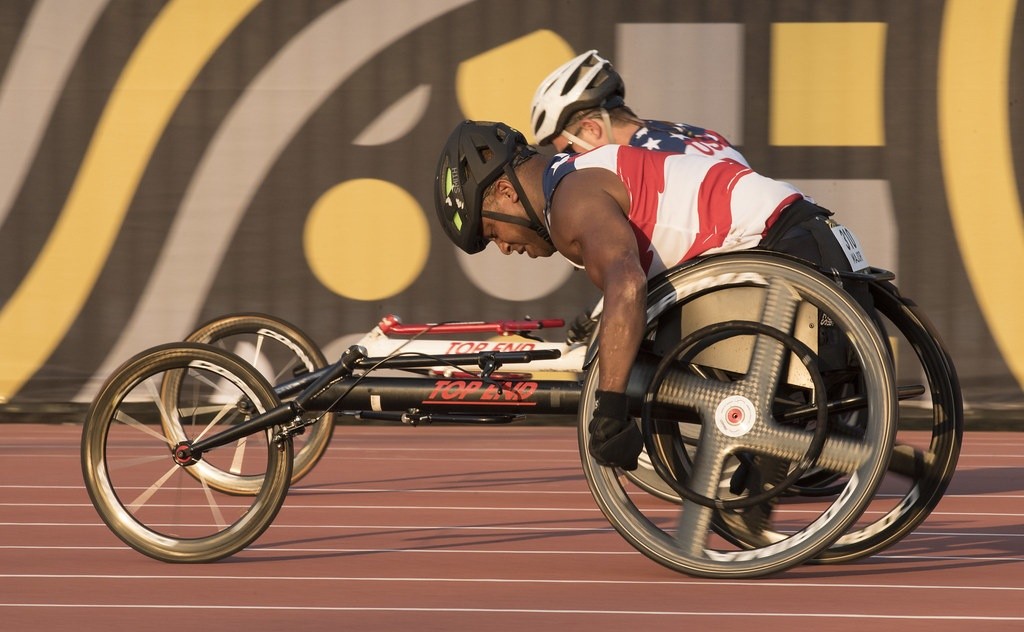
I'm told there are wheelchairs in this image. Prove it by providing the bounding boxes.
[76,248,965,580]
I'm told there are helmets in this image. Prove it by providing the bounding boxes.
[531,49,625,146]
[434,119,526,255]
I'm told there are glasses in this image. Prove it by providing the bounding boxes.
[560,126,582,155]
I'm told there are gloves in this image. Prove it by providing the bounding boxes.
[588,389,642,470]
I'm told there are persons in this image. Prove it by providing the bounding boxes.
[529,48,749,167]
[434,119,870,472]
